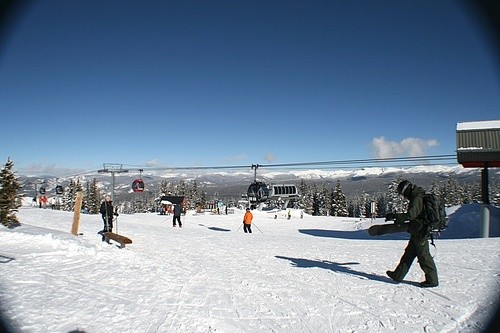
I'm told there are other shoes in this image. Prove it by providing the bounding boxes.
[386,271,402,283]
[421,281,438,287]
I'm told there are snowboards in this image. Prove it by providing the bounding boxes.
[368,219,450,237]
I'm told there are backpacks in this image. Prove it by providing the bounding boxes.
[423,193,449,232]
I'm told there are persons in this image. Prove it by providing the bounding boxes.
[33,196,47,209]
[300,211,303,218]
[173,203,182,228]
[100,195,119,241]
[160,205,168,215]
[243,208,253,233]
[385,180,438,287]
[52,198,56,209]
[288,210,291,219]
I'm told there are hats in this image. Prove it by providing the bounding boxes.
[397,181,412,195]
[246,208,250,211]
